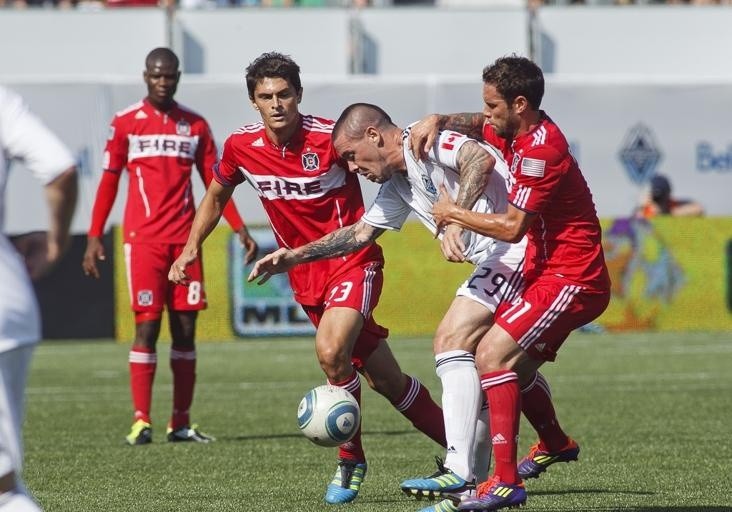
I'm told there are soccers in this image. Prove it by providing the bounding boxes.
[298,385,361,446]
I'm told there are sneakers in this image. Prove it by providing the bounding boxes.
[324,459,368,504]
[167,423,215,443]
[517,437,579,479]
[400,455,526,511]
[125,420,152,446]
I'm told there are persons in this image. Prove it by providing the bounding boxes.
[0,82,78,512]
[168,52,446,505]
[406,56,610,511]
[83,47,258,443]
[632,175,704,219]
[247,104,531,500]
